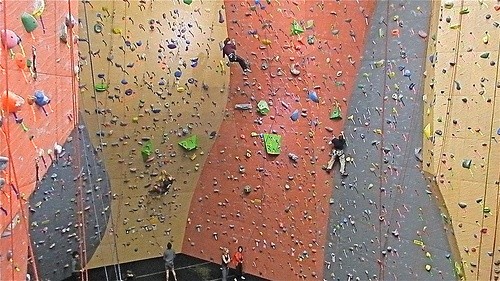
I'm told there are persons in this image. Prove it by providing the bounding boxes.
[71,250,82,281]
[222,37,252,75]
[327,131,348,176]
[148,170,174,195]
[163,242,177,281]
[221,247,230,281]
[233,246,245,281]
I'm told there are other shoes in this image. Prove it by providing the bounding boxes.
[241,276,245,279]
[244,69,252,73]
[341,171,348,176]
[234,278,237,281]
